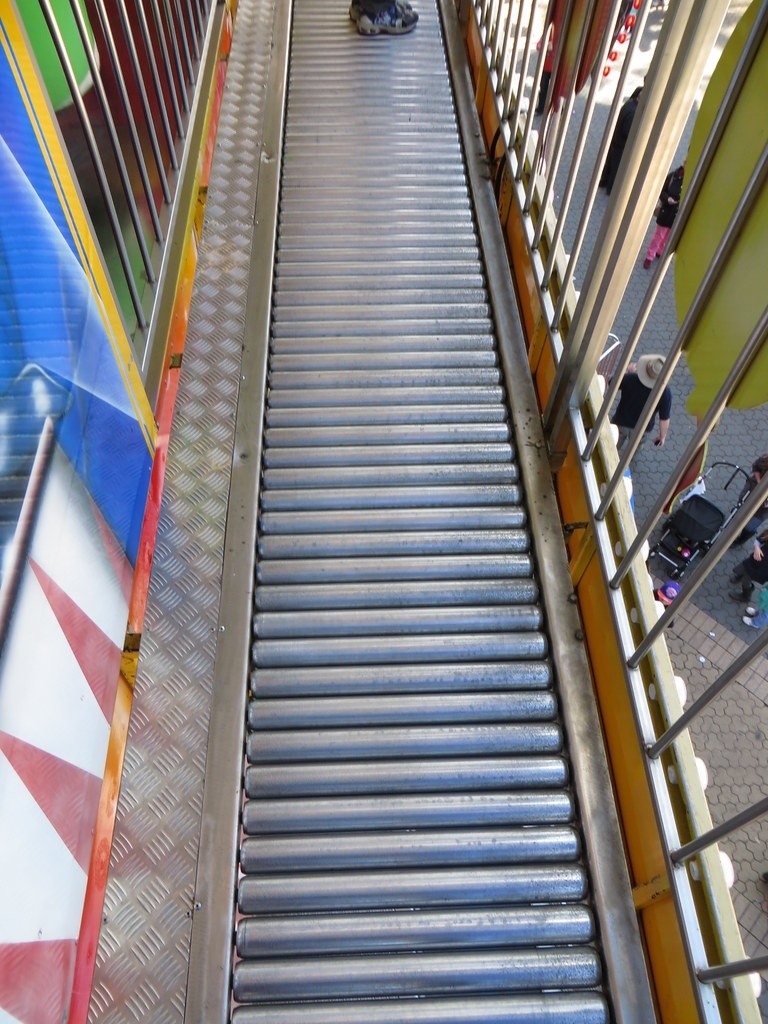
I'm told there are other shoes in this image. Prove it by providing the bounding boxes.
[745,607,757,616]
[742,615,759,629]
[535,108,544,114]
[655,251,660,257]
[643,258,653,269]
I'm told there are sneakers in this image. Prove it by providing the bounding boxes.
[349,0,412,23]
[356,8,419,36]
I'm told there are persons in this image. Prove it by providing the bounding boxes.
[653,580,681,628]
[728,454,768,630]
[643,144,691,270]
[598,75,647,196]
[608,354,672,474]
[349,0,419,36]
[534,21,559,117]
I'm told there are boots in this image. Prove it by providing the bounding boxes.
[729,583,754,603]
[731,573,744,584]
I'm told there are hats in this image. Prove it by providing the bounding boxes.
[657,580,682,605]
[637,354,667,390]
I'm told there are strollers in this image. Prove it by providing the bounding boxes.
[649,461,756,586]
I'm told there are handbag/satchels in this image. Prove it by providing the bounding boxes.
[536,22,554,53]
[653,199,664,217]
